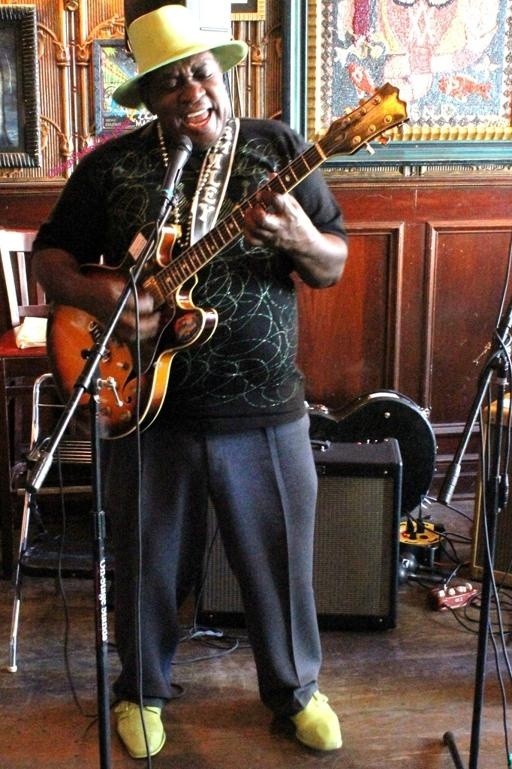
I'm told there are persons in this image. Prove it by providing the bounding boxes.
[31,3,349,760]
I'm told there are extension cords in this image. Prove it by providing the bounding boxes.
[428,585,478,611]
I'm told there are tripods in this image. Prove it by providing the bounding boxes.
[433,302,511,769]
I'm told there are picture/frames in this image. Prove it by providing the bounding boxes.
[0,2,45,169]
[285,0,512,181]
[88,37,160,135]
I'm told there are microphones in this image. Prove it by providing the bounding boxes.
[156,134,194,222]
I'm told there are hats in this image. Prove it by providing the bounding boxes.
[109,5,250,110]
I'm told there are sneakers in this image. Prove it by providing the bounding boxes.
[287,690,345,751]
[115,701,167,759]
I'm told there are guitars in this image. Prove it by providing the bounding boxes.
[46,82,410,441]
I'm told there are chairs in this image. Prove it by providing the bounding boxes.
[1,226,116,613]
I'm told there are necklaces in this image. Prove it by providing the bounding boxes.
[157,116,228,248]
